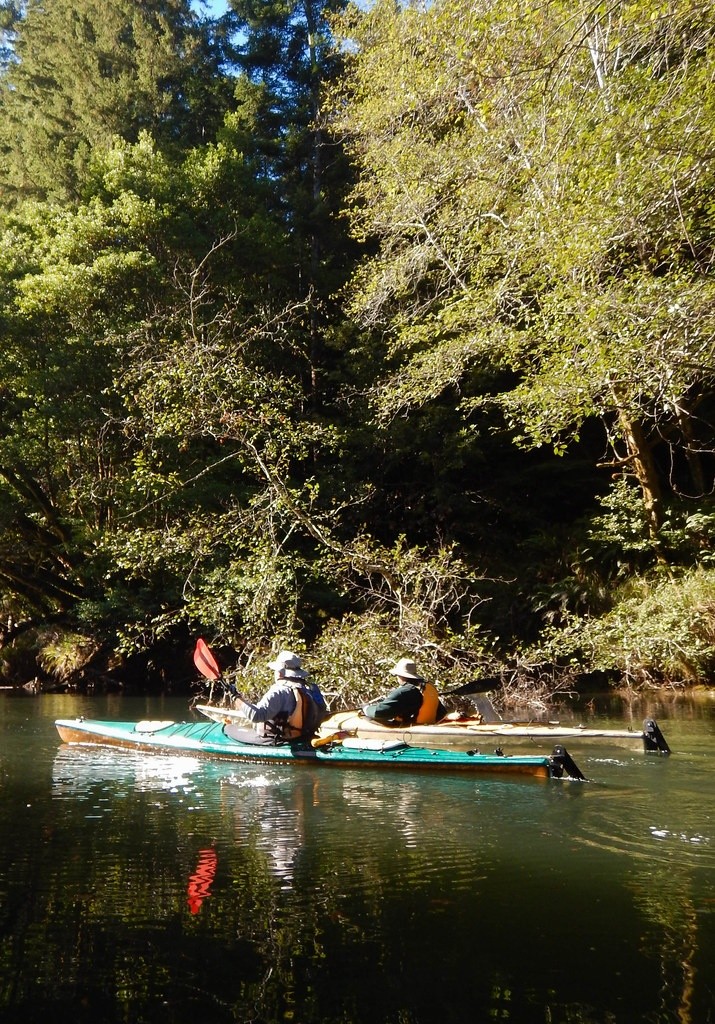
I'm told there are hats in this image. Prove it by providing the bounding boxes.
[389,659,424,679]
[267,651,309,678]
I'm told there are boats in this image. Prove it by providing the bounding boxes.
[54,716,586,780]
[193,702,673,756]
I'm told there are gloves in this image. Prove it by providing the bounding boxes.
[225,685,241,701]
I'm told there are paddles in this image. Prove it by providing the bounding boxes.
[325,678,502,716]
[192,639,261,714]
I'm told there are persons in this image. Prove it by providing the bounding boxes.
[224,651,319,747]
[359,658,447,727]
